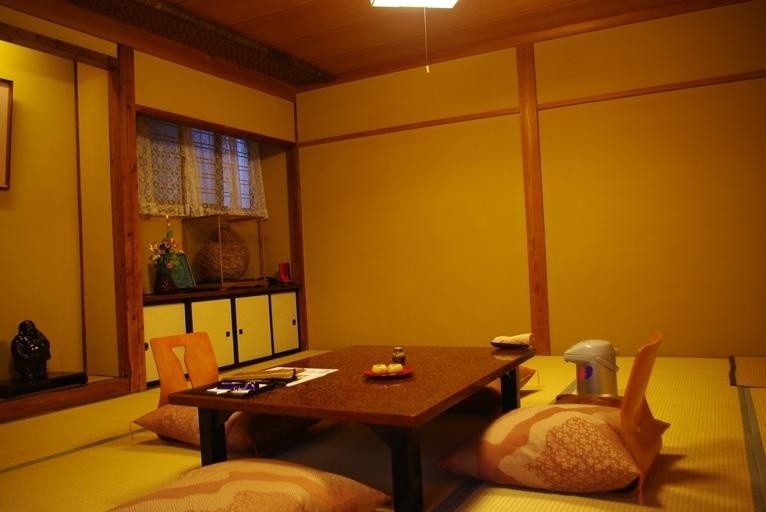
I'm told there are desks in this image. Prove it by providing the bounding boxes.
[162,343,541,510]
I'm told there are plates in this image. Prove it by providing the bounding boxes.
[489,341,532,350]
[361,364,414,378]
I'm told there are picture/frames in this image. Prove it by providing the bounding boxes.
[0,77,16,194]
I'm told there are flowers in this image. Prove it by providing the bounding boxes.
[145,210,184,277]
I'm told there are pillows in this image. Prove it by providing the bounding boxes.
[436,400,676,496]
[101,454,393,511]
[133,399,325,455]
[455,356,539,413]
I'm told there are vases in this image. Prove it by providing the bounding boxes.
[153,273,177,294]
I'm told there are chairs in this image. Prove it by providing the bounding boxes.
[147,327,220,447]
[552,325,666,506]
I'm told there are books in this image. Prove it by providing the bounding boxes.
[223,368,305,380]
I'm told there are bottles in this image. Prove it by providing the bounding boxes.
[390,347,405,363]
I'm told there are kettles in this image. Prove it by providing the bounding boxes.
[562,338,618,396]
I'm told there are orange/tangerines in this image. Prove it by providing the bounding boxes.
[388,364,403,374]
[372,364,387,374]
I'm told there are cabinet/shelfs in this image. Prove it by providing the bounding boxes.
[269,285,301,355]
[186,291,273,372]
[141,298,190,389]
